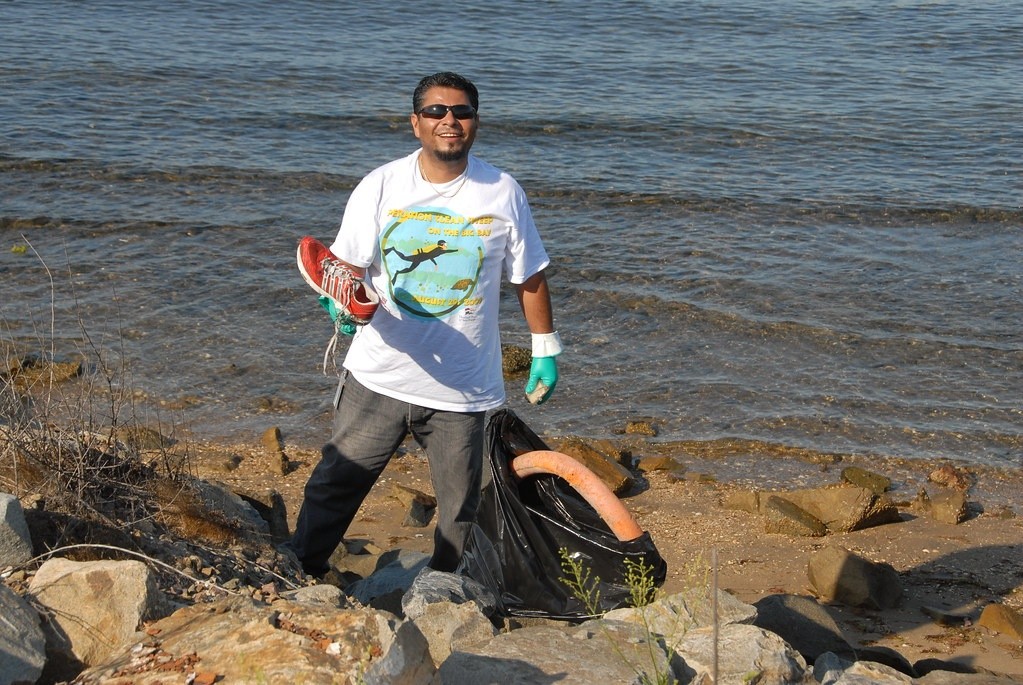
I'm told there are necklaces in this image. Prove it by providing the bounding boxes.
[418,153,469,198]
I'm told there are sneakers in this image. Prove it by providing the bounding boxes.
[297,236,379,326]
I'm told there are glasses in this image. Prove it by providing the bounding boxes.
[417,104,478,121]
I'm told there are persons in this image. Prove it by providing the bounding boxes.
[289,71,566,578]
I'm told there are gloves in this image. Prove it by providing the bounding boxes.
[319,296,357,335]
[525,331,565,406]
